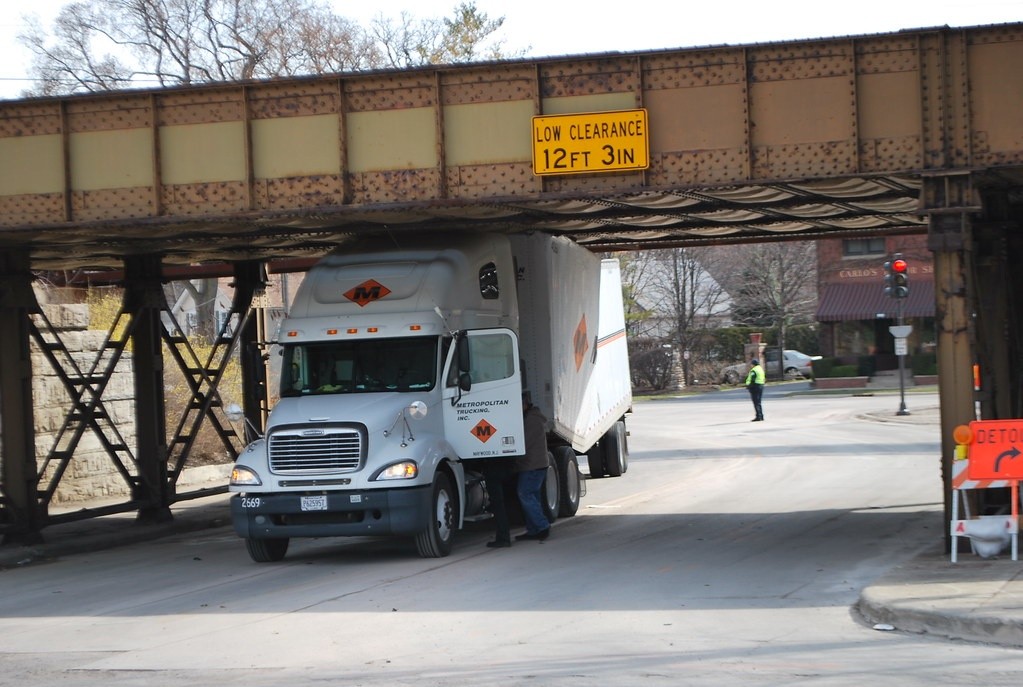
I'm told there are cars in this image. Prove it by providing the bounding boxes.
[720,348,823,386]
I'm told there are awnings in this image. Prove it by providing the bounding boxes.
[817,278,936,322]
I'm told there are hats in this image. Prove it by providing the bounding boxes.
[752,360,759,365]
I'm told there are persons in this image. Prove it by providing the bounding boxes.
[514,390,551,540]
[747,360,766,422]
[483,457,511,547]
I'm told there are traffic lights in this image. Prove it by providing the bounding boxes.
[882,261,895,297]
[893,260,909,298]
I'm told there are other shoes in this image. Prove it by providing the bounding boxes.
[515,533,537,540]
[752,418,763,421]
[487,541,510,547]
[537,526,550,540]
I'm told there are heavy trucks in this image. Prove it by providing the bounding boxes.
[222,225,636,564]
